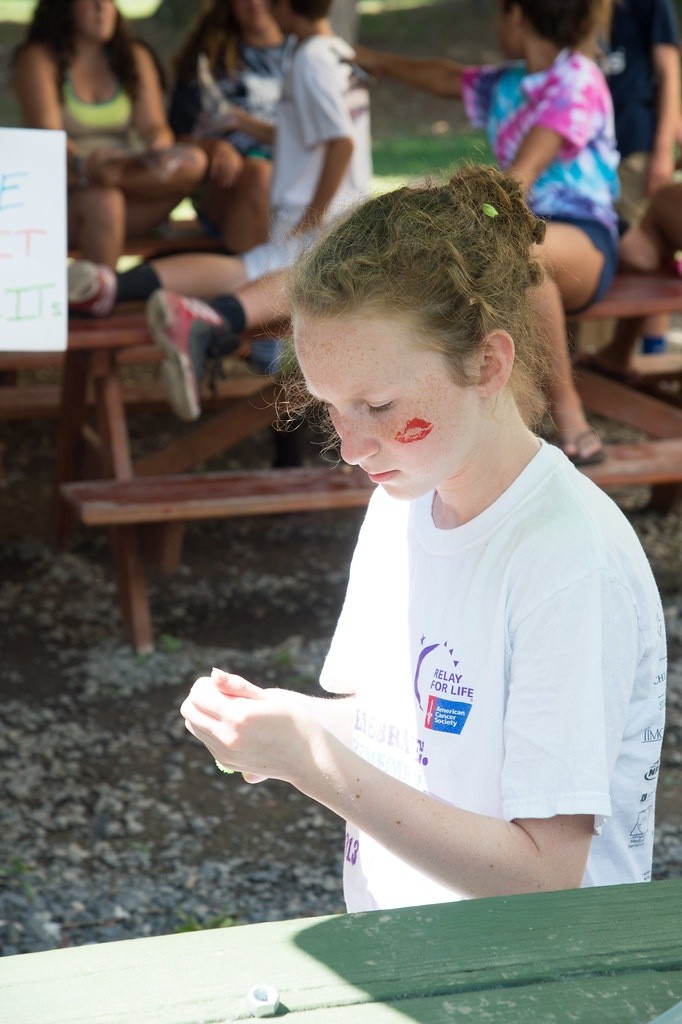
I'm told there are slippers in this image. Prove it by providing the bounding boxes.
[563,430,605,468]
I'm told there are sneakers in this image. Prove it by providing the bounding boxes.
[147,287,239,422]
[68,256,118,318]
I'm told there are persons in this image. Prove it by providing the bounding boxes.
[178,165,668,915]
[572,0,681,379]
[341,0,623,469]
[66,0,378,431]
[8,2,208,274]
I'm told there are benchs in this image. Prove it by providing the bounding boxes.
[1,215,682,658]
